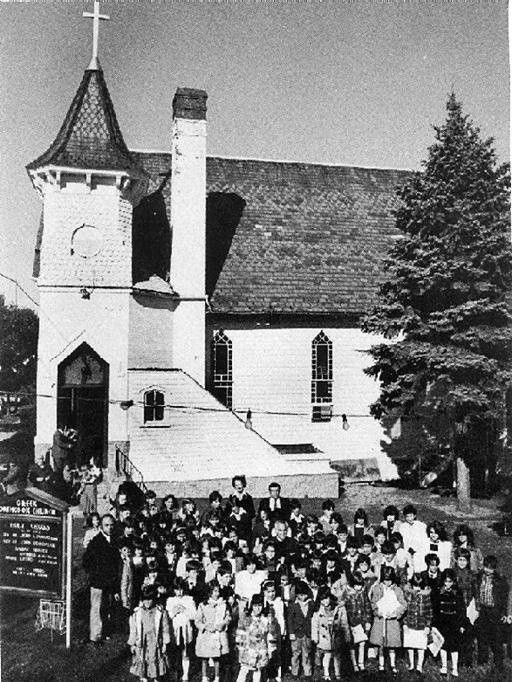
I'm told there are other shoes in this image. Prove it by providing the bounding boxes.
[181,662,282,682]
[352,663,423,675]
[438,661,503,677]
[290,662,343,682]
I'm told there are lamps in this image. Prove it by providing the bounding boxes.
[339,412,350,432]
[243,409,252,428]
